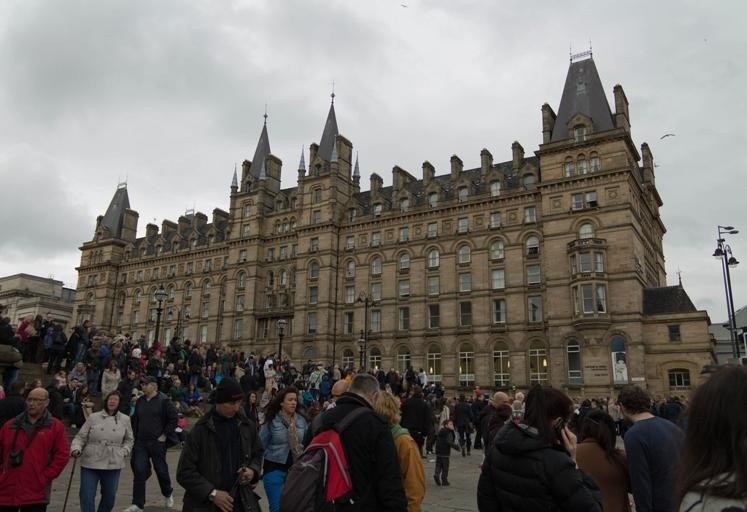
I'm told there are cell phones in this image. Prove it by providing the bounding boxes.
[552,417,569,444]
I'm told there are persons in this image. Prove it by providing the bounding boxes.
[0,312,746,511]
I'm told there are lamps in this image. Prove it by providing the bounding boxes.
[543,357,547,367]
[430,366,433,373]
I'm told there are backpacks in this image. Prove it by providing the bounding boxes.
[279,406,378,512]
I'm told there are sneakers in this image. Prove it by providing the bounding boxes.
[166,493,173,508]
[123,504,144,512]
[442,481,449,485]
[434,474,441,485]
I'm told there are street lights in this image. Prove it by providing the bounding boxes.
[276,319,288,372]
[354,290,377,373]
[711,225,740,358]
[154,284,169,351]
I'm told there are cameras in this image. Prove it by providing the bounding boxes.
[8,448,24,467]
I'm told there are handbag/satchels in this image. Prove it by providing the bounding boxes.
[215,464,262,512]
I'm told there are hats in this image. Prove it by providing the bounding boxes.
[216,377,245,403]
[139,376,158,385]
